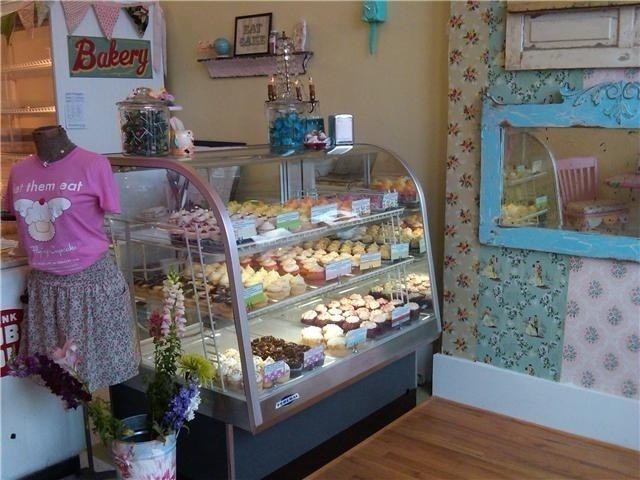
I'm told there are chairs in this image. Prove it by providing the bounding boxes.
[555,156,630,234]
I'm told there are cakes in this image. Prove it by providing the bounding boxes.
[133,265,162,289]
[501,161,539,223]
[369,272,431,305]
[348,175,425,249]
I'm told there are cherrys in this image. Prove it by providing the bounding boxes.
[118,107,171,156]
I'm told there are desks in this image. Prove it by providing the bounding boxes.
[603,173,640,188]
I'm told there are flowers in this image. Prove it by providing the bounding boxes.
[6,266,215,446]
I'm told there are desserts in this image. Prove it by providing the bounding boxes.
[166,190,422,396]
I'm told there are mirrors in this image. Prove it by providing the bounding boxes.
[478,79,640,263]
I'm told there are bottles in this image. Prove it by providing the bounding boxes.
[266,96,323,152]
[268,30,279,57]
[117,86,172,160]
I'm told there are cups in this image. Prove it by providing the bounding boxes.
[292,24,310,52]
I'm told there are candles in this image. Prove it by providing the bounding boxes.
[263,32,320,114]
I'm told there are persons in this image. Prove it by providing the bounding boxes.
[5,125,139,387]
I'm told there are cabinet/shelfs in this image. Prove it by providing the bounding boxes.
[0,1,173,220]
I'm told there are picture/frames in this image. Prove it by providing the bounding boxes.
[233,12,272,57]
[177,140,247,211]
